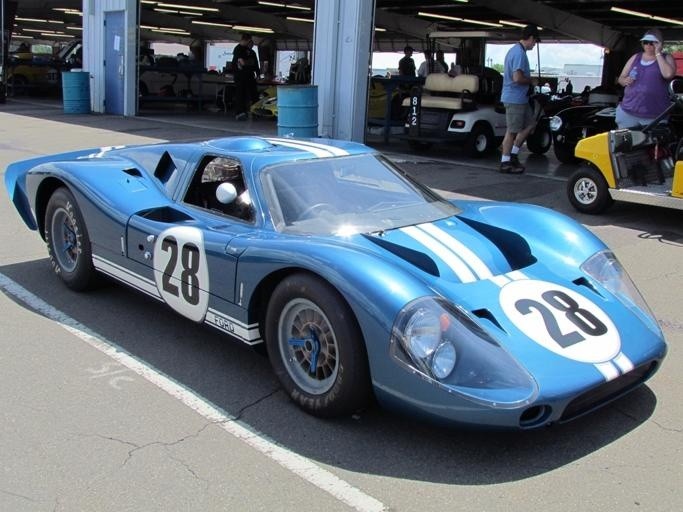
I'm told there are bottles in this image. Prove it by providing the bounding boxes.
[630,65,639,81]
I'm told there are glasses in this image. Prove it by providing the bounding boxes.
[642,41,657,46]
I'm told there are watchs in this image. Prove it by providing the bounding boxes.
[655,52,663,57]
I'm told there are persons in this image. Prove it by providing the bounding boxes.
[614,29,677,129]
[397,46,415,77]
[384,72,390,78]
[232,33,253,121]
[244,40,260,114]
[418,49,446,78]
[499,29,541,175]
[435,50,448,73]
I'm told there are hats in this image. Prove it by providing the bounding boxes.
[639,34,660,42]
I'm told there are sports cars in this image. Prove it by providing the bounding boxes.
[251,74,411,121]
[2,134,668,436]
[397,73,682,161]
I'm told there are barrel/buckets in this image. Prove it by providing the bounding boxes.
[60,70,91,113]
[276,83,318,137]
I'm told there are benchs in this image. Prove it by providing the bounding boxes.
[401,73,479,128]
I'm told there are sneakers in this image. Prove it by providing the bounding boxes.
[499,152,526,174]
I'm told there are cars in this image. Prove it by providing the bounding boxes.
[4,50,67,91]
